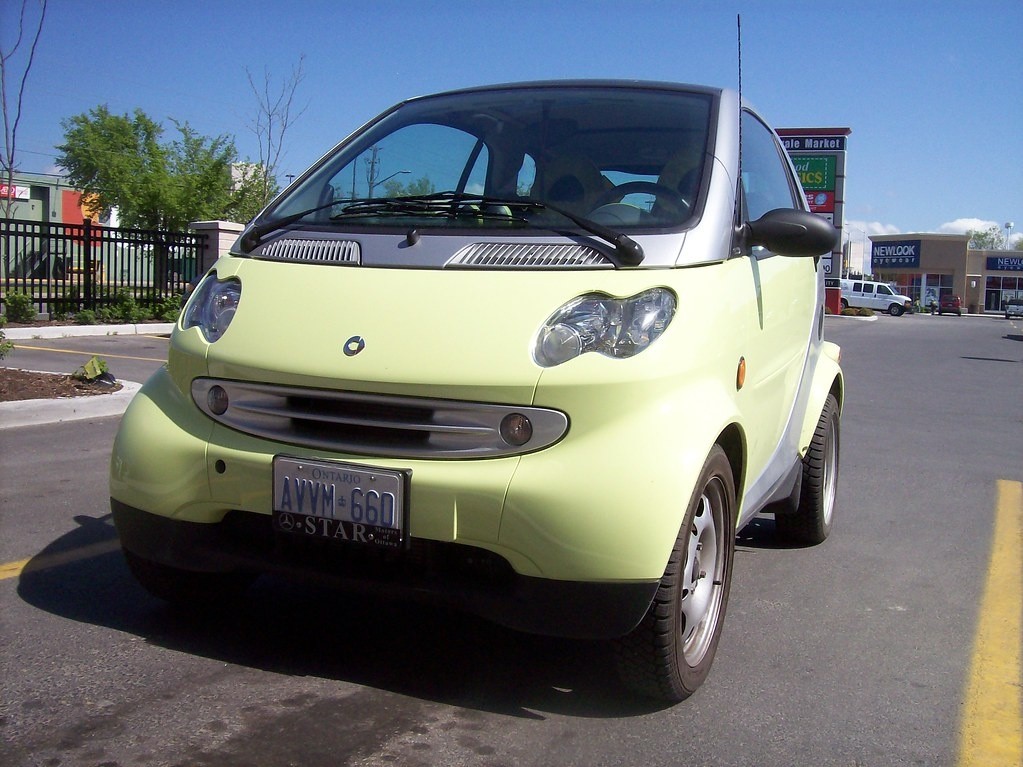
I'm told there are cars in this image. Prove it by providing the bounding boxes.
[938,294,961,316]
[106,78,845,707]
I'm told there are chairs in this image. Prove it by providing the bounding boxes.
[529,151,603,211]
[650,155,704,218]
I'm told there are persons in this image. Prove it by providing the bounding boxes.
[929,299,937,315]
[655,143,704,214]
[913,296,922,313]
[529,144,604,224]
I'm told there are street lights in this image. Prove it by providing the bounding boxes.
[645,200,655,210]
[844,223,866,281]
[285,174,296,184]
[368,170,412,199]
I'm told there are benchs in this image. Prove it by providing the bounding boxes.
[57,256,100,275]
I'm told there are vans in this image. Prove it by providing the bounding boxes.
[840,279,913,317]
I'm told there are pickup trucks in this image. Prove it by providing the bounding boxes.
[1004,299,1023,319]
